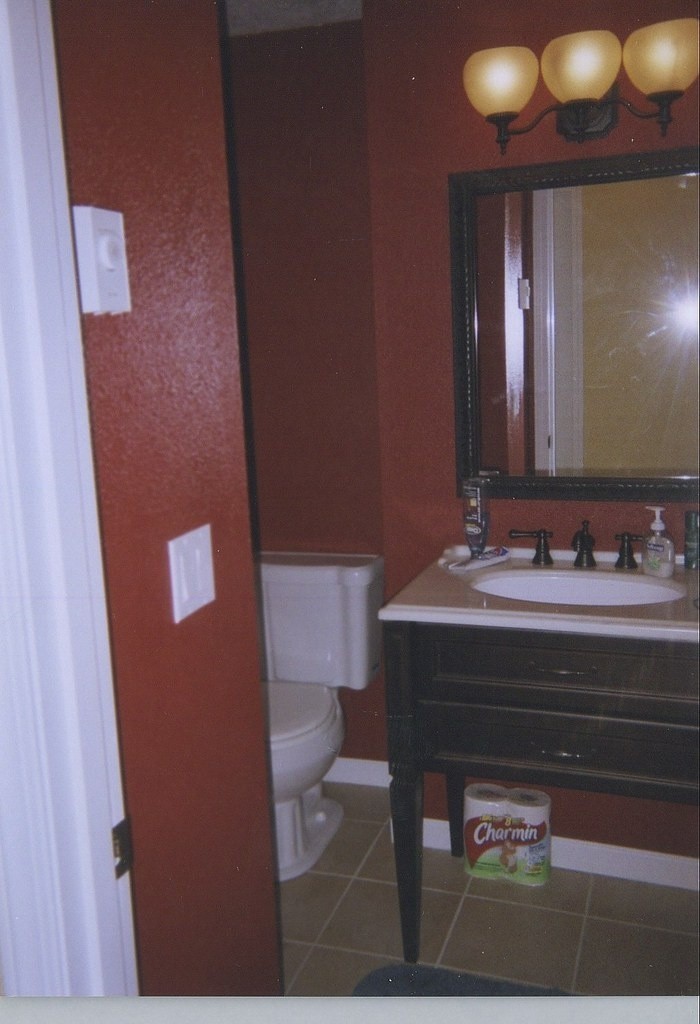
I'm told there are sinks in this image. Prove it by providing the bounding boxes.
[464,567,688,607]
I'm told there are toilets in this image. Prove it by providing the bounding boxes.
[256,550,387,885]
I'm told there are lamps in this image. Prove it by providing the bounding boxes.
[459,16,700,156]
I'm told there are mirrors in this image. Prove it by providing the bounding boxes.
[446,147,700,507]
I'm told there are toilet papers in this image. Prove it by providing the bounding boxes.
[463,782,551,886]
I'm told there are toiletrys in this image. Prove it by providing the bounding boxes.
[642,506,676,578]
[462,484,488,556]
[449,545,511,571]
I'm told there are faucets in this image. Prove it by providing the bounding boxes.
[571,520,596,567]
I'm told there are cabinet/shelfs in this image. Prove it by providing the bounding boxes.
[381,616,699,967]
